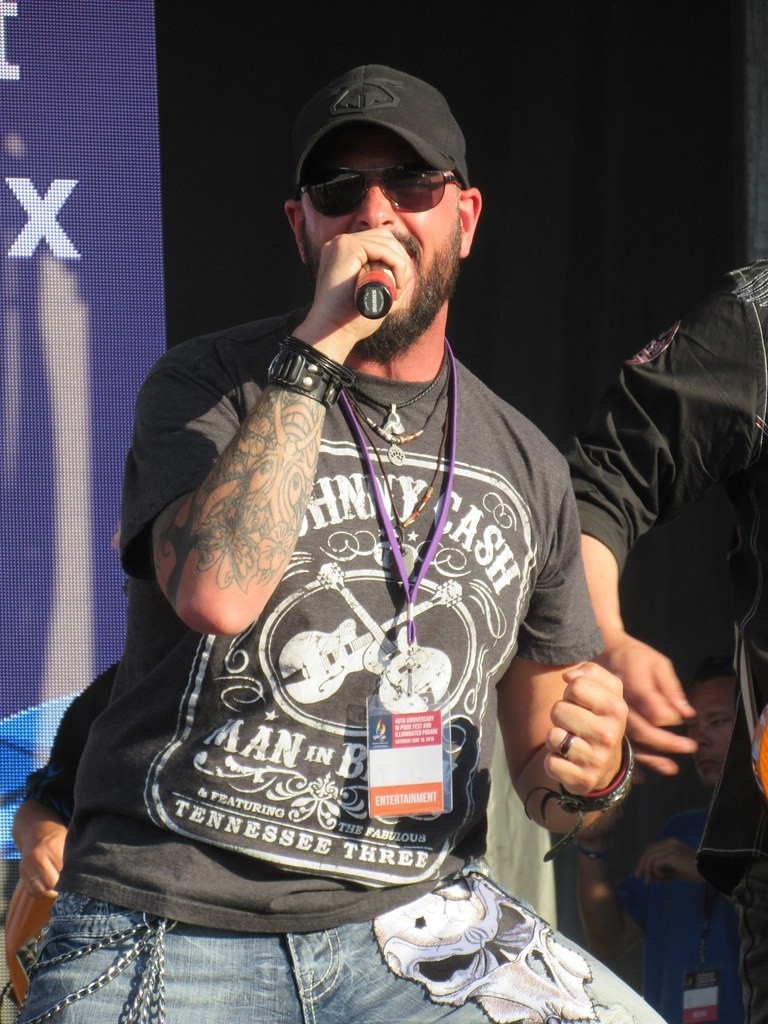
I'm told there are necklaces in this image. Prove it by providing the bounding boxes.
[340,343,450,556]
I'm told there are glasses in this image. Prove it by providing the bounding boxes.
[296,162,464,219]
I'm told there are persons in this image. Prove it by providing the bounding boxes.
[562,253,768,1024]
[12,64,673,1024]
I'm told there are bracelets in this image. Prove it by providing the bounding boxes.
[267,335,356,408]
[525,733,634,862]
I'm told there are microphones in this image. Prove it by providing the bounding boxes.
[353,260,396,320]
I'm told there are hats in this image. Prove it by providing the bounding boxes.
[292,64,470,207]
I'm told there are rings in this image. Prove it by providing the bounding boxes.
[559,732,577,759]
[29,875,40,882]
